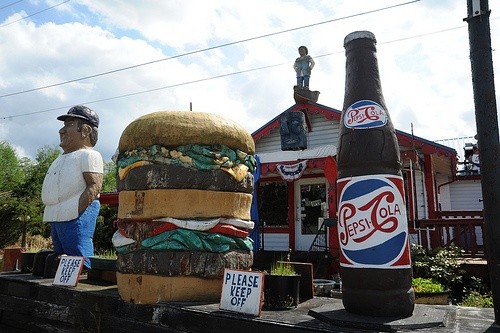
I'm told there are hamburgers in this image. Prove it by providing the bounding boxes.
[112,110,256,306]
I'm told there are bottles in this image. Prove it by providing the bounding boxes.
[335,30,415,317]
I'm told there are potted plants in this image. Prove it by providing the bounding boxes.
[413,278,454,304]
[86,248,118,271]
[264,255,301,311]
[428,243,468,301]
[20,248,39,273]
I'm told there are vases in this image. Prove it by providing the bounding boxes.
[314,279,336,296]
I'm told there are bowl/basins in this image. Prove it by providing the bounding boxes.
[313,279,336,296]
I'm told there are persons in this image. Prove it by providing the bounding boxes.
[293,46,315,90]
[41,106,104,276]
